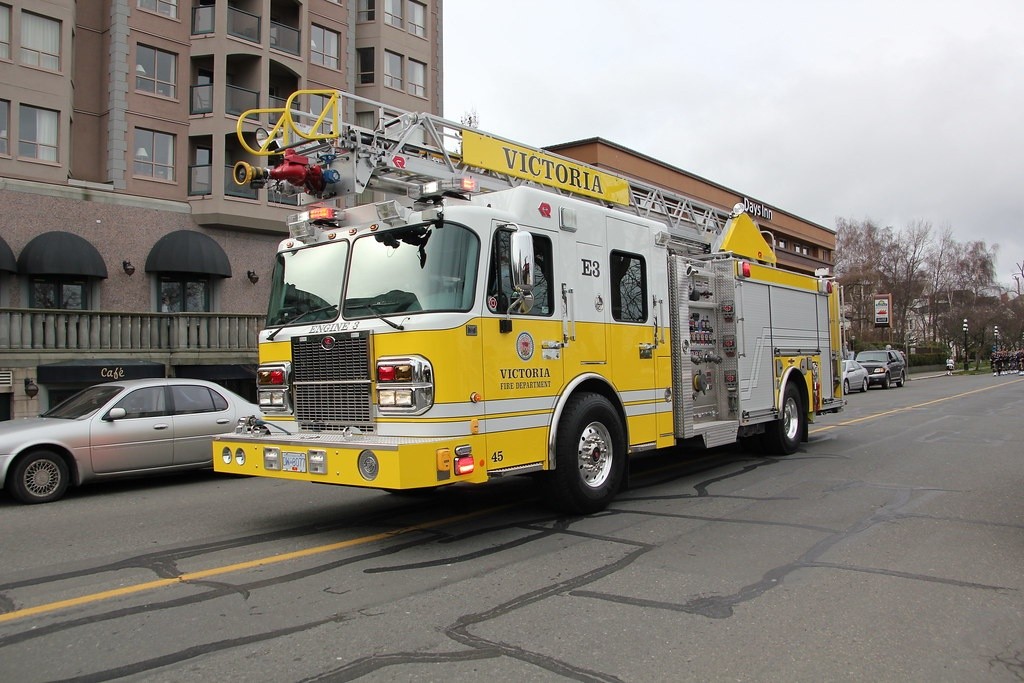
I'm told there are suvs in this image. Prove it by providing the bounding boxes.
[855,345,906,389]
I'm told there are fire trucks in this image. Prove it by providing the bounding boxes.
[210,89,848,520]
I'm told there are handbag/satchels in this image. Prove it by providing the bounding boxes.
[949,365,954,369]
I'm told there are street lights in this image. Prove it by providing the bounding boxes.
[993,324,1000,357]
[963,318,969,371]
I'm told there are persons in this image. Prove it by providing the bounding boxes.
[990,346,1024,376]
[946,356,954,376]
[900,351,907,361]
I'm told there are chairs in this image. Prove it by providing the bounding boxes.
[270,27,277,45]
[196,93,209,110]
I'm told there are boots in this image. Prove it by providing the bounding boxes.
[993,370,1024,376]
[945,370,952,376]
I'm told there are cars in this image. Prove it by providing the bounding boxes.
[841,359,869,395]
[0,378,266,505]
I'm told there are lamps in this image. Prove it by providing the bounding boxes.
[247,270,259,285]
[23,378,39,399]
[0,130,7,154]
[310,39,317,62]
[135,64,147,90]
[123,259,135,277]
[136,148,149,171]
